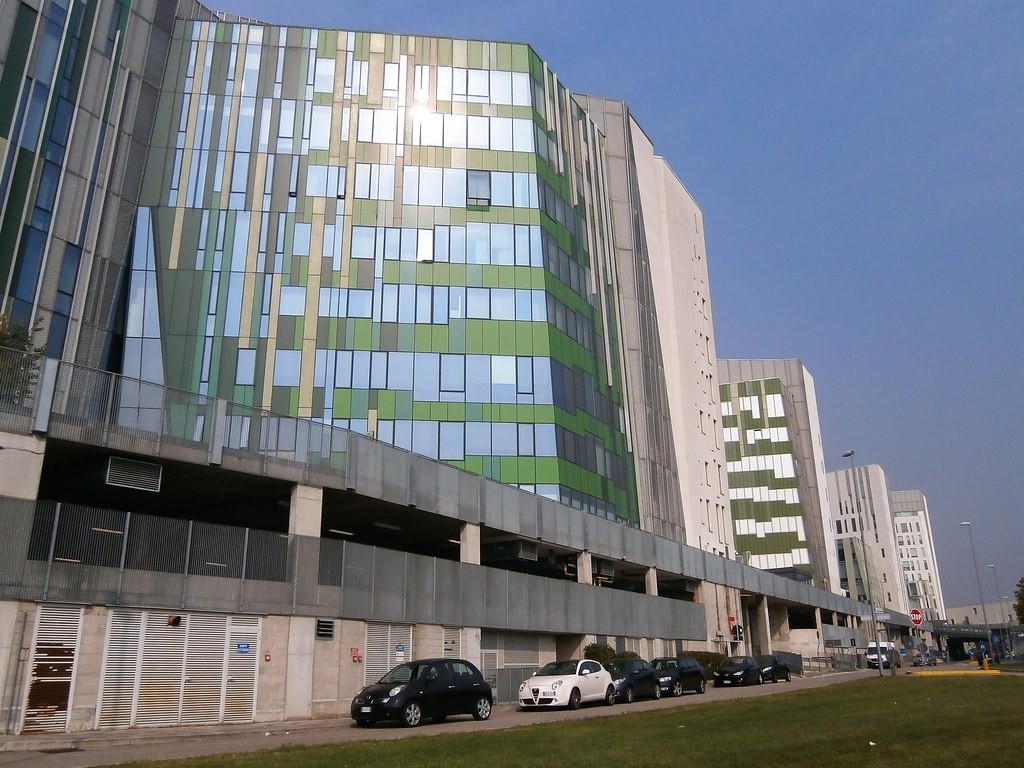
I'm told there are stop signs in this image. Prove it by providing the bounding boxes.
[909,608,924,626]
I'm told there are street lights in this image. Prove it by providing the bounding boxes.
[987,563,1007,663]
[960,522,996,665]
[999,596,1012,662]
[842,448,884,676]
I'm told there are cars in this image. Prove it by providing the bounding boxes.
[646,655,707,698]
[755,655,792,682]
[350,657,493,728]
[713,656,766,688]
[913,652,938,667]
[599,657,662,704]
[518,659,616,711]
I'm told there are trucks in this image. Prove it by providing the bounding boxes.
[864,641,896,669]
